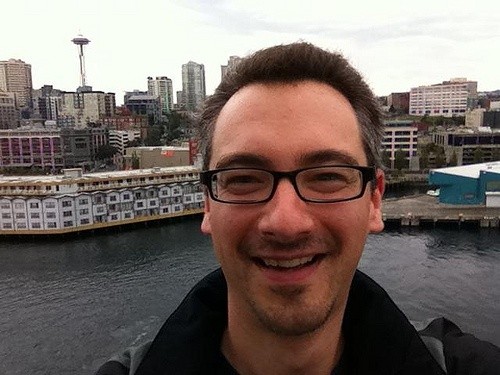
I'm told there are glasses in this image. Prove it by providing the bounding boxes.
[199,165,377,204]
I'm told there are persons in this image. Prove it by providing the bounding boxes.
[92,45,499,375]
